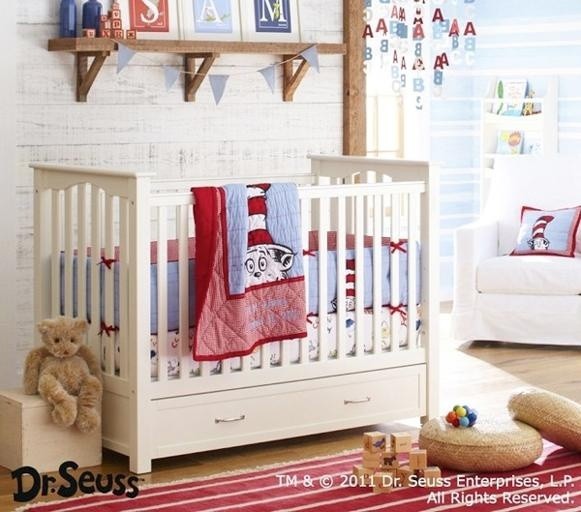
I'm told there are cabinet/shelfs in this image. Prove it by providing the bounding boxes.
[479,75,559,214]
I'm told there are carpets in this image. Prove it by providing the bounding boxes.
[14,436,581,512]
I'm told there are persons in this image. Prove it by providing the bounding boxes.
[24,317,103,434]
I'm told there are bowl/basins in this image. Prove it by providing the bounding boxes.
[441,416,479,429]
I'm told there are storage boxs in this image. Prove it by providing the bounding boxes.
[0,385,103,476]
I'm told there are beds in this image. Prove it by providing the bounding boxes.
[29,152,439,474]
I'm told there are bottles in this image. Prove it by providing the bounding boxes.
[60,0,78,38]
[82,1,104,29]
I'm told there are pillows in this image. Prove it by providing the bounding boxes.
[418,410,544,471]
[507,385,581,453]
[509,204,581,258]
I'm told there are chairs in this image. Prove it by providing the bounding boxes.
[453,149,581,346]
